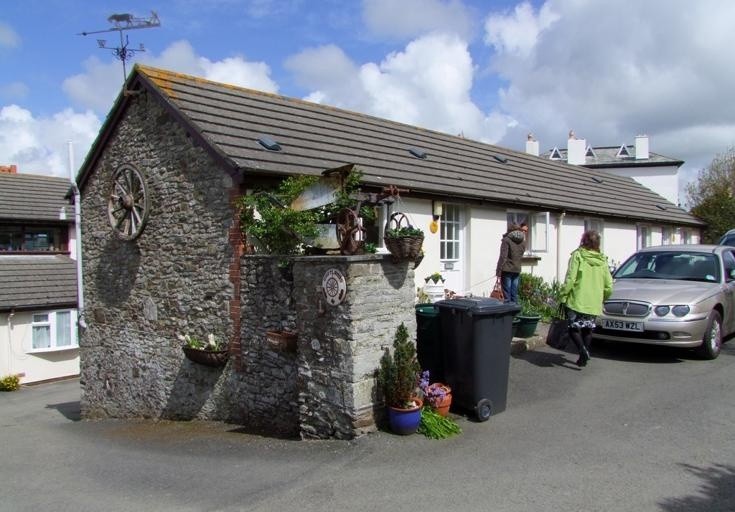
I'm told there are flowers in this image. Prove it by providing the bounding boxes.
[177,333,221,352]
[418,370,447,409]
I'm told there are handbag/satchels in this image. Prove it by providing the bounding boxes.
[546,303,569,351]
[491,276,504,301]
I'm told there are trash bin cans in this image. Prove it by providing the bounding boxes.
[415,298,523,423]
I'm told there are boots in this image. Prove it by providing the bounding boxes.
[576,345,590,367]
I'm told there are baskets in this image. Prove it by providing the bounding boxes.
[384,236,424,257]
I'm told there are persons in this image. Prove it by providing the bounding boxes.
[496,222,527,304]
[557,229,615,366]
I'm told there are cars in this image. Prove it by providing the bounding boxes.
[591,229,734,360]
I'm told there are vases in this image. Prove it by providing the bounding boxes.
[425,386,452,418]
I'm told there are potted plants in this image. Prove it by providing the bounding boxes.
[512,299,542,337]
[374,321,424,435]
[384,225,425,259]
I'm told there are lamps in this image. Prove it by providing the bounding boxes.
[432,198,443,221]
